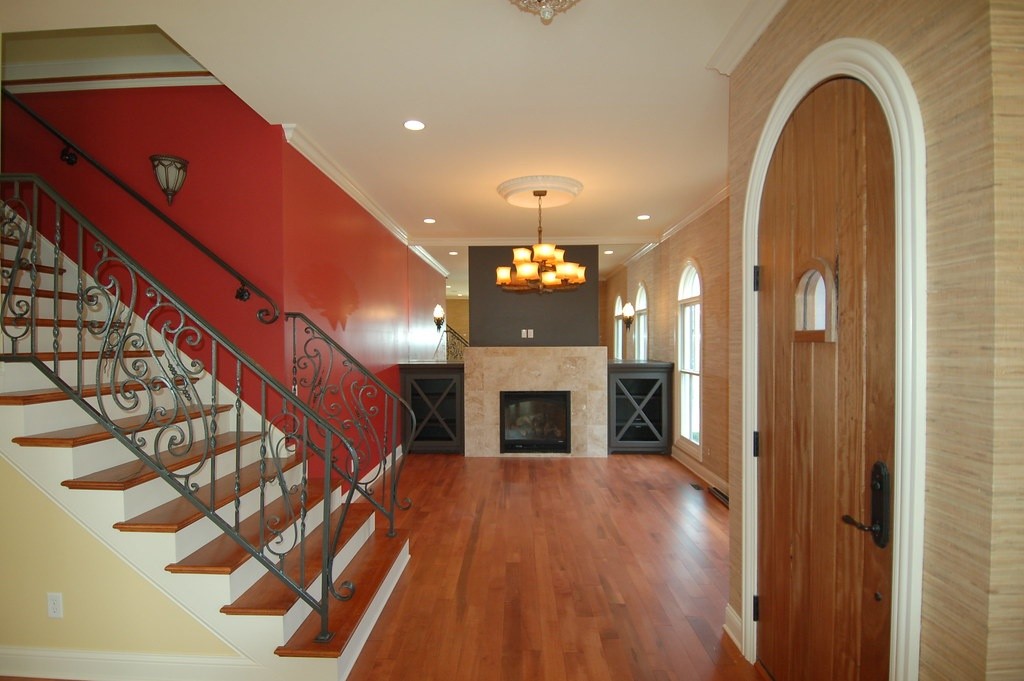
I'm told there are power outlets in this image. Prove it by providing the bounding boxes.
[48,593,64,618]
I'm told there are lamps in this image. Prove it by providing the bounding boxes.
[510,0,580,23]
[496,189,588,296]
[149,154,189,206]
[623,302,635,332]
[432,303,445,332]
